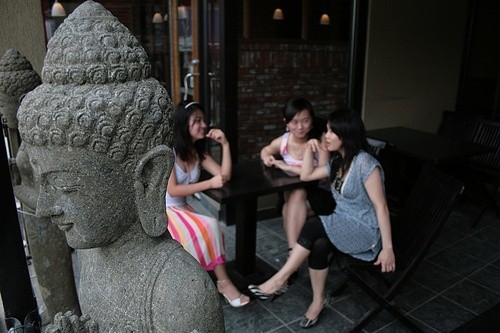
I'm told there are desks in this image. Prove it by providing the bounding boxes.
[362,125,492,166]
[199,158,323,307]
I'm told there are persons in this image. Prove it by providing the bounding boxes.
[164,100,250,307]
[16,0,225,333]
[259,98,331,255]
[248,113,396,327]
[0,49,82,320]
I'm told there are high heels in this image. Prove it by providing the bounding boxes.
[216,280,250,307]
[298,293,330,327]
[248,283,289,303]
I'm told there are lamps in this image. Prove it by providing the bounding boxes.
[152,10,163,23]
[320,11,330,24]
[49,0,67,17]
[273,6,283,21]
[178,6,189,20]
[164,13,169,22]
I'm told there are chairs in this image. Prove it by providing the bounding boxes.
[462,119,500,229]
[435,108,483,142]
[328,164,467,333]
